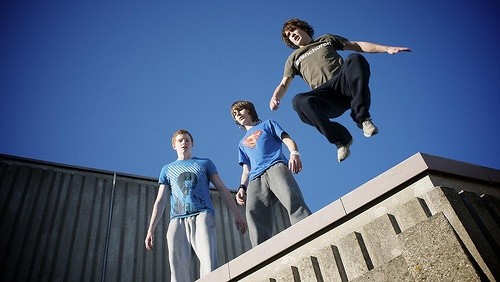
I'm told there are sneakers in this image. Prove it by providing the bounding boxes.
[337,138,353,162]
[362,119,378,137]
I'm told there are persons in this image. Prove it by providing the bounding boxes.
[270,20,412,163]
[145,130,247,282]
[230,101,314,248]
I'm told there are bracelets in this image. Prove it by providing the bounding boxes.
[238,184,247,193]
[289,151,300,157]
